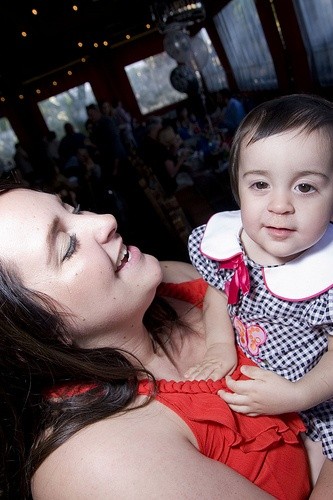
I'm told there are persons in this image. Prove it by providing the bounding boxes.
[182,95,333,488]
[0,187,333,500]
[13,88,247,212]
